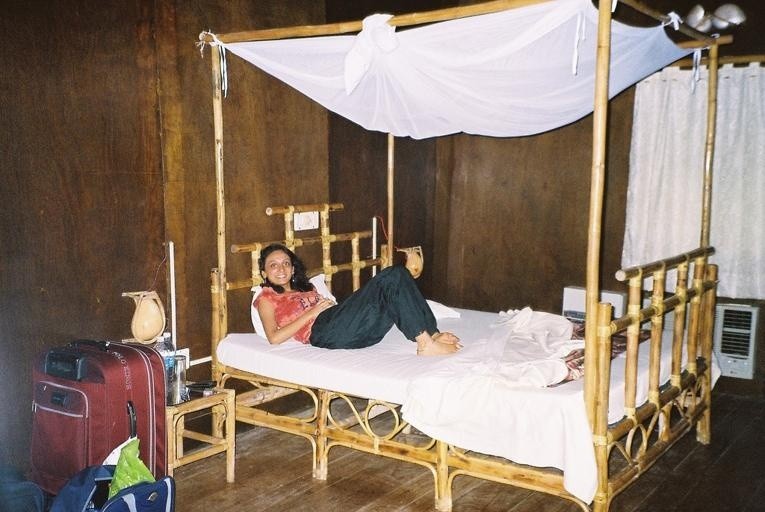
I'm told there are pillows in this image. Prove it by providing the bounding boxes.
[249,274,337,339]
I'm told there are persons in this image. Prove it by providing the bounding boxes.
[254,243,463,357]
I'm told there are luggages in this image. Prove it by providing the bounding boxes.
[30,339,168,497]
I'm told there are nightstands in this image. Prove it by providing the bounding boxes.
[166,388,236,484]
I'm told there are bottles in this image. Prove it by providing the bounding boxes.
[165,331,178,408]
[153,338,171,403]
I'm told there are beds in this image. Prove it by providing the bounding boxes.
[199,263,732,512]
